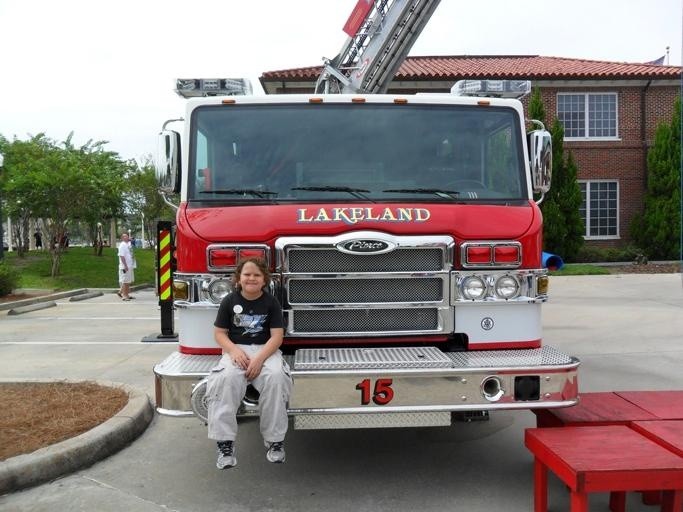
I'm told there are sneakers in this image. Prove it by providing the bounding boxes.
[216,439,237,470]
[263,437,286,464]
[117,292,130,302]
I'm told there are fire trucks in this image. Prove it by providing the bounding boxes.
[153,0,580,437]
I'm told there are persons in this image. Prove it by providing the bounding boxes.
[207,256,294,470]
[117,232,136,301]
[34,228,42,250]
[51,228,69,250]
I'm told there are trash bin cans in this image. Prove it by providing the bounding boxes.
[542,251,563,272]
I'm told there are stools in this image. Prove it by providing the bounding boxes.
[513,383,682,512]
